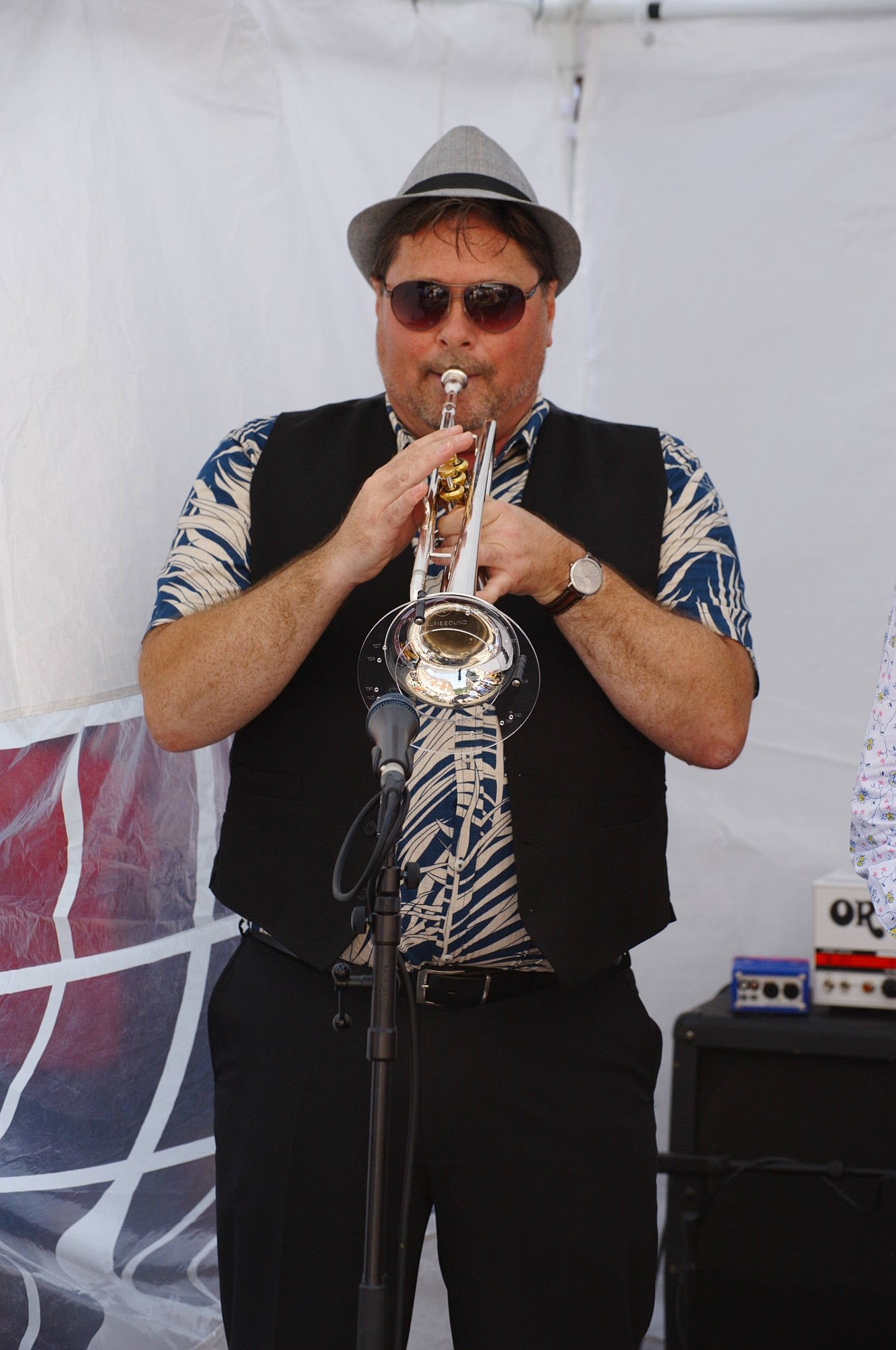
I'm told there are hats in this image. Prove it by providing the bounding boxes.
[348,126,581,299]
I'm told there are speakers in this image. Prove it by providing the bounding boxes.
[657,979,895,1350]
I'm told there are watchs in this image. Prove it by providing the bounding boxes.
[539,550,608,618]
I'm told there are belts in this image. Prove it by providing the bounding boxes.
[250,930,554,1009]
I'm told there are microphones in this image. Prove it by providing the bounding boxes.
[364,692,421,798]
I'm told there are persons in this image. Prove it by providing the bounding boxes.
[134,124,762,1349]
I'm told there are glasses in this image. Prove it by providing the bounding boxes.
[377,276,546,333]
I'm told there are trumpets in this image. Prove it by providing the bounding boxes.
[346,365,541,754]
